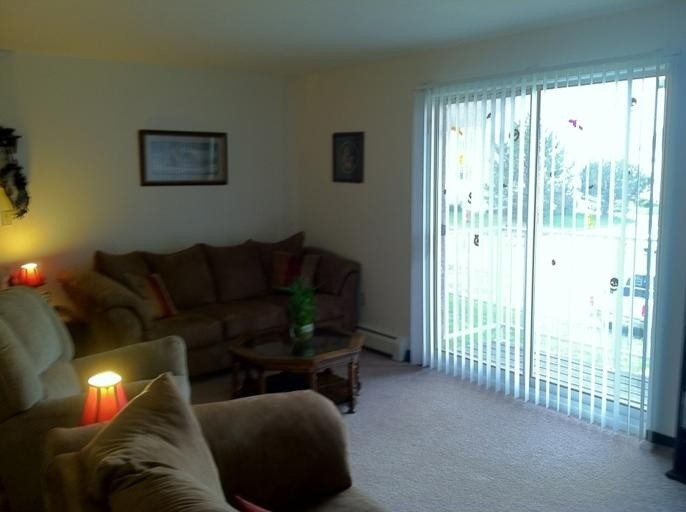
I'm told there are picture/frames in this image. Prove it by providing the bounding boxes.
[137,129,228,186]
[332,131,366,184]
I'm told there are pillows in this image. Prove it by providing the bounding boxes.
[121,269,179,319]
[77,375,236,512]
[258,243,319,305]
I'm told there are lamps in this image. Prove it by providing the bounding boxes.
[79,374,132,427]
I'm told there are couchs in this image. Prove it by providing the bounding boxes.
[0,286,193,430]
[62,232,362,378]
[37,387,388,511]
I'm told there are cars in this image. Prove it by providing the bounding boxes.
[588,238,658,353]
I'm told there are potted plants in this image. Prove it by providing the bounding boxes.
[275,279,315,346]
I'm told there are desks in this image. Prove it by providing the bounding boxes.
[222,323,360,413]
